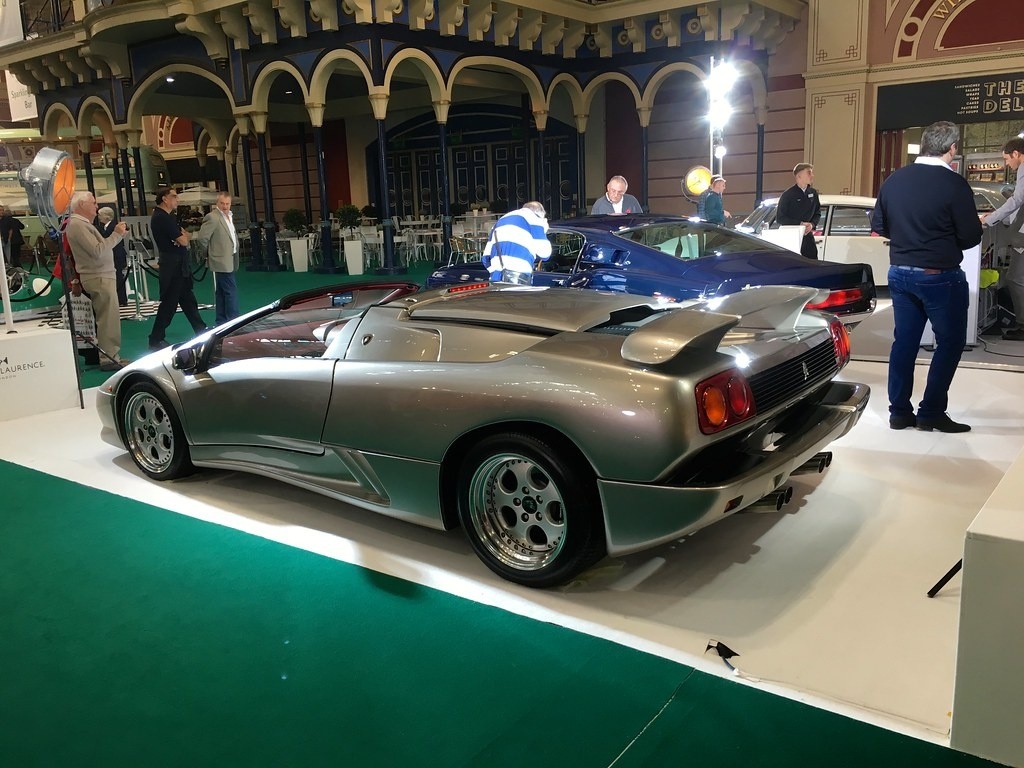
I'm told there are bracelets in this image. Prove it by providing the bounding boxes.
[70,279,80,284]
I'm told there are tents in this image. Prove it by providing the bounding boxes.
[177,186,243,216]
[95,187,156,207]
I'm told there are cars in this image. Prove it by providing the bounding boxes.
[654,194,889,286]
[968,181,1016,267]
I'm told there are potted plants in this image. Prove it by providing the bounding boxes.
[471,203,480,216]
[360,204,378,249]
[282,208,309,272]
[480,201,490,214]
[417,210,427,221]
[335,205,365,275]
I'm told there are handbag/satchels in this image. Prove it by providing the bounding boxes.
[58,292,98,349]
[502,270,531,285]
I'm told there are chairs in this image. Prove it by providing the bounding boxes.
[238,214,502,272]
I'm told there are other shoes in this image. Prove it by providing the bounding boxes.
[99,361,131,370]
[1002,329,1024,340]
[149,340,170,349]
[916,409,971,432]
[890,412,918,430]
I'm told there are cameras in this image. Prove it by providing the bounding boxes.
[124,223,131,231]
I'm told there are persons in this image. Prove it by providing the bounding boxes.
[705,177,732,246]
[148,187,212,350]
[591,176,643,244]
[96,208,128,307]
[482,201,551,284]
[52,218,99,364]
[0,206,25,269]
[198,191,239,325]
[66,191,129,371]
[980,138,1024,341]
[179,206,203,225]
[871,121,984,432]
[698,174,721,220]
[776,163,821,260]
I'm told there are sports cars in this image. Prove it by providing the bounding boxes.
[425,211,877,336]
[97,278,873,589]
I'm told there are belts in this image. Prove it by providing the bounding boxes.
[894,265,926,271]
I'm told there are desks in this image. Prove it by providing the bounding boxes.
[401,219,439,262]
[489,212,507,221]
[453,215,496,264]
